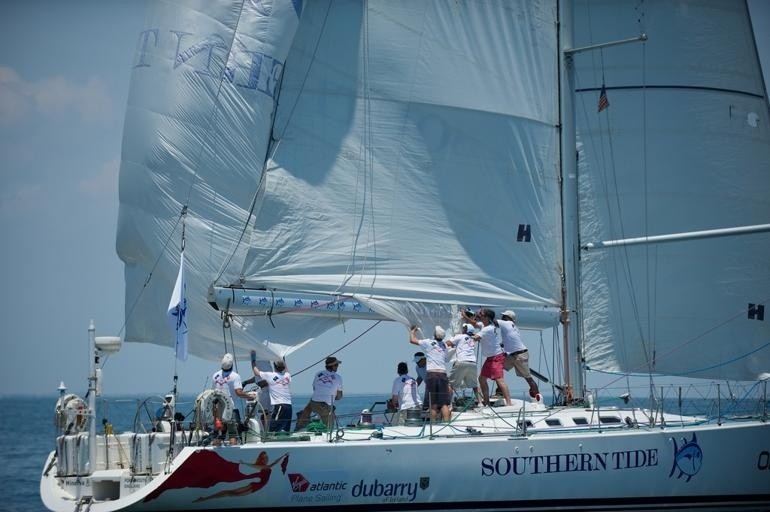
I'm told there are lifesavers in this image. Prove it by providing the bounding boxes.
[193,390,235,430]
[56,393,88,433]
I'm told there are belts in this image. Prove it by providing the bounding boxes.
[510,349,527,355]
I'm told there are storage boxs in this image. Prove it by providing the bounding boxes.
[93,470,122,501]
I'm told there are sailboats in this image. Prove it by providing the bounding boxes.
[39,2,770,512]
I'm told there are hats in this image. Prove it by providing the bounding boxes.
[412,306,516,363]
[221,353,342,370]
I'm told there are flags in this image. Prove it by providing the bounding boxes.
[166,249,189,363]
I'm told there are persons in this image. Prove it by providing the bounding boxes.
[251,350,293,440]
[154,396,173,431]
[390,307,541,425]
[102,418,113,435]
[294,356,346,435]
[191,450,290,504]
[210,353,257,445]
